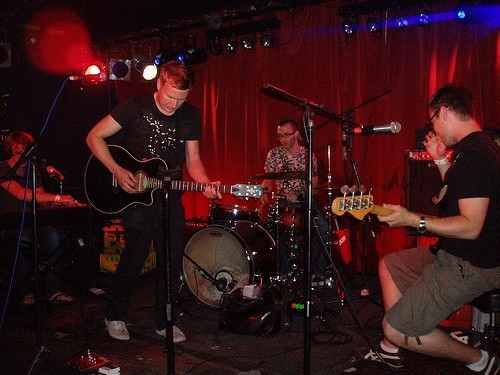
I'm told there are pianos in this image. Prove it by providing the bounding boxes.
[0,204,94,307]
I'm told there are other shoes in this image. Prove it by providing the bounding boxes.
[311,275,331,286]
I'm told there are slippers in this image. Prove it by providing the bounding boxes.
[46,294,75,306]
[23,296,36,305]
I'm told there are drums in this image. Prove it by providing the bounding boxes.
[180,213,281,310]
[204,199,261,228]
[270,205,305,235]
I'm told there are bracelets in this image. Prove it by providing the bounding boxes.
[55,195,61,202]
[434,157,448,165]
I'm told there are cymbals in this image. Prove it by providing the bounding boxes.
[313,183,341,192]
[251,169,318,181]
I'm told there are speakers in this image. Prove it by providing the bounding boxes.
[38,84,118,192]
[404,148,458,234]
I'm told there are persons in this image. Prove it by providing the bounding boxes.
[355,84,500,375]
[257,118,329,287]
[85,60,223,343]
[0,130,105,305]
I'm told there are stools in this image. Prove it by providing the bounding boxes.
[473,288,500,354]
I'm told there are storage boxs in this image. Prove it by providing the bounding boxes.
[101,219,158,275]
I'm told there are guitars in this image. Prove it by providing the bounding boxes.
[330,184,439,230]
[83,143,265,216]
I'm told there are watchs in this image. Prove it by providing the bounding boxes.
[417,217,427,233]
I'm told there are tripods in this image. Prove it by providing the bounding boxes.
[323,136,383,307]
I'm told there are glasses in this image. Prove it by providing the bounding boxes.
[428,105,448,127]
[277,130,296,138]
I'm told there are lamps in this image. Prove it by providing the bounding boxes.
[339,0,497,35]
[0,24,16,71]
[69,0,276,91]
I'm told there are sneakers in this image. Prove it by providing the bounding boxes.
[105,318,130,340]
[464,351,500,375]
[355,342,404,368]
[155,324,186,343]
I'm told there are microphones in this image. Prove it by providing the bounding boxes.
[215,277,227,291]
[68,71,107,81]
[348,121,401,133]
